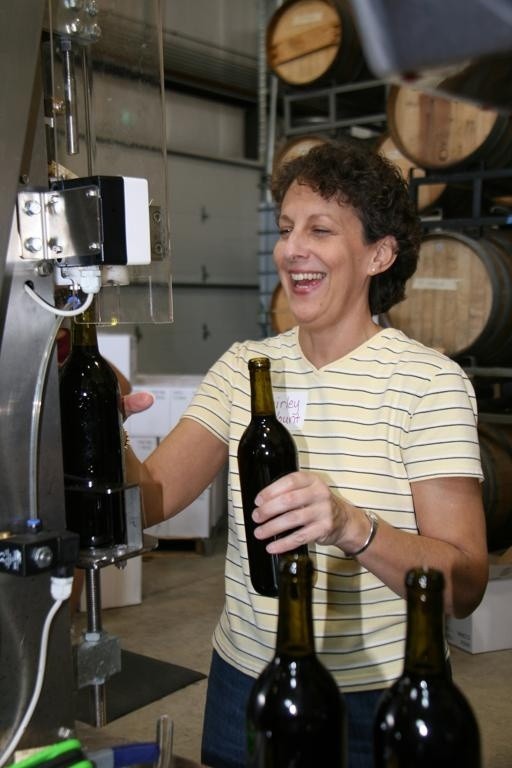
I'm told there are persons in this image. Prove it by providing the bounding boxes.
[54,140,489,768]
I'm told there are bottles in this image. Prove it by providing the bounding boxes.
[370,566,485,768]
[236,356,314,596]
[56,288,131,551]
[243,548,349,767]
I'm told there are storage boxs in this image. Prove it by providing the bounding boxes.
[441,562,512,653]
[95,331,228,538]
[70,554,155,612]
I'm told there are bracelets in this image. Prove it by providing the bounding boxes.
[123,427,129,452]
[344,508,380,557]
